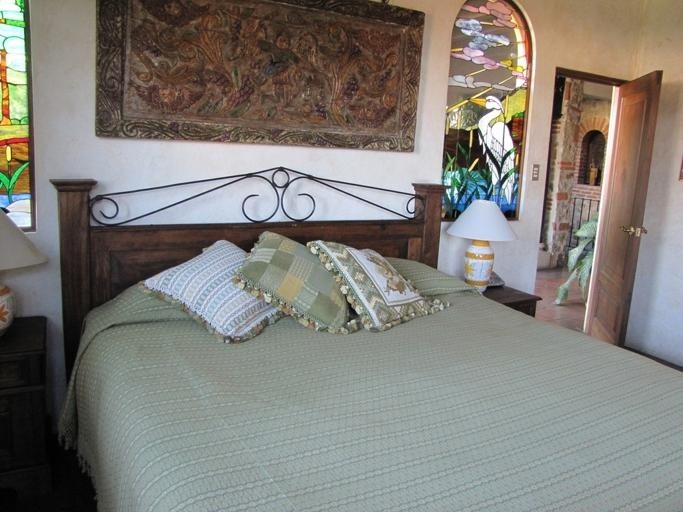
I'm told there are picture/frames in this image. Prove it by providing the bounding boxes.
[94,0,425,154]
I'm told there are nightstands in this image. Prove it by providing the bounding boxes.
[476,282,543,319]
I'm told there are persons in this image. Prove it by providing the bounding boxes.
[590,158,599,186]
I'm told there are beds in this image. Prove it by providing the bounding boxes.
[48,165,682,511]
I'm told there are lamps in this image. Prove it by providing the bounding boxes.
[0,204,50,338]
[444,200,518,299]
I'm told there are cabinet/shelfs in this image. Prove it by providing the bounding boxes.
[0,315,53,512]
